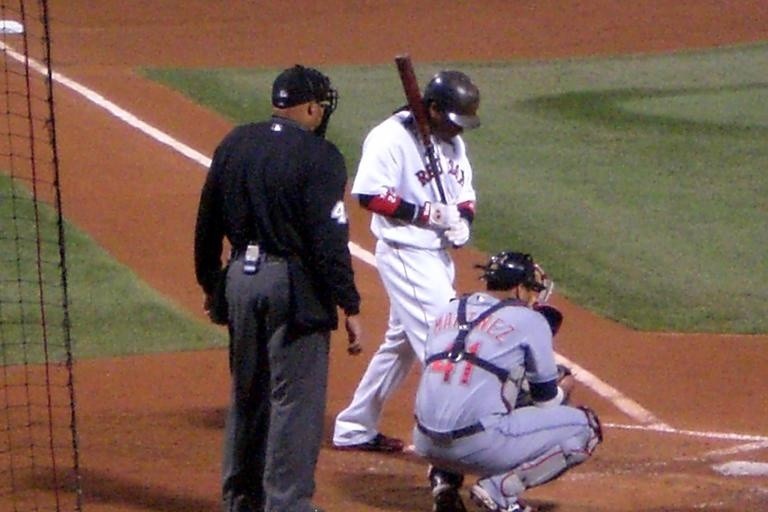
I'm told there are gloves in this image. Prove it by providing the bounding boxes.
[413,201,461,230]
[443,216,469,247]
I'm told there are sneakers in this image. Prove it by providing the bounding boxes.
[431,474,467,511]
[469,484,534,512]
[332,433,404,453]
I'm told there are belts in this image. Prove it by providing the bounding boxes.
[231,251,284,265]
[414,416,484,443]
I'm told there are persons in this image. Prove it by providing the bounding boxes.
[333,69,481,452]
[194,66,364,512]
[412,252,602,512]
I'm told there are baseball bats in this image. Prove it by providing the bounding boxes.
[394,52,462,249]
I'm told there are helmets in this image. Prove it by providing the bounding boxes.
[272,63,338,141]
[425,68,481,130]
[475,250,553,307]
